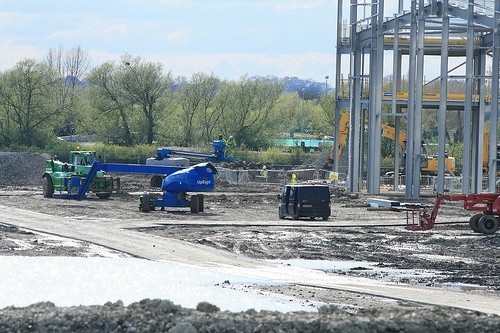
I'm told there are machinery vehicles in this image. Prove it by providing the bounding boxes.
[153,140,236,162]
[44,149,121,197]
[405,191,500,234]
[322,111,461,176]
[53,160,217,212]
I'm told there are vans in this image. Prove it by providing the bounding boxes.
[277,185,330,219]
